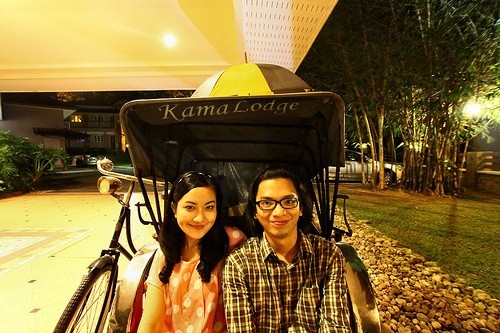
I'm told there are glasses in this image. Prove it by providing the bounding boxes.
[255,197,301,211]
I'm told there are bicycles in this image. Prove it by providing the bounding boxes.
[51,157,171,333]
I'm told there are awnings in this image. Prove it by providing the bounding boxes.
[33,128,84,136]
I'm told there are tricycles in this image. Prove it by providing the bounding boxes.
[107,51,385,333]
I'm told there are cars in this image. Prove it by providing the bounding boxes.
[313,149,404,186]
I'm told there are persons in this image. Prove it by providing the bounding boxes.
[221,170,353,333]
[136,170,229,333]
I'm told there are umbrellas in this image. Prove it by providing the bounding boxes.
[192,52,312,97]
